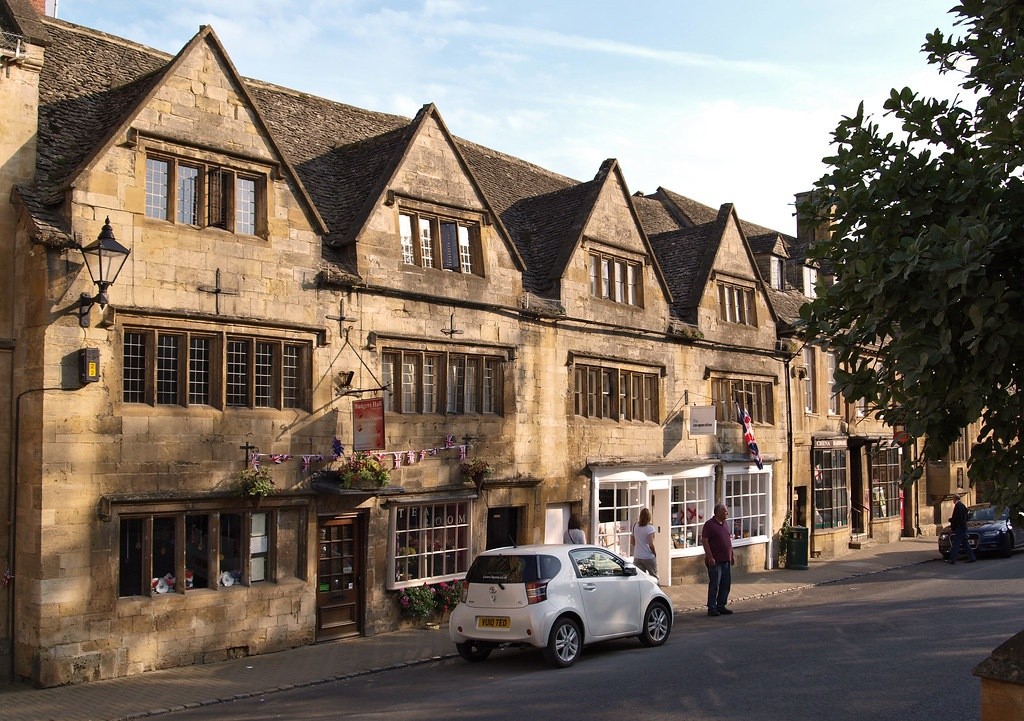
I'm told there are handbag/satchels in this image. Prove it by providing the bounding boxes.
[630,523,637,556]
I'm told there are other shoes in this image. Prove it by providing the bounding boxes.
[944,558,955,565]
[708,608,720,616]
[716,607,733,615]
[964,558,976,563]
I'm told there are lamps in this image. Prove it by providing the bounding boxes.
[77,215,131,327]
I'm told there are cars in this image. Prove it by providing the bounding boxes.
[938,502,1024,559]
[448,544,674,668]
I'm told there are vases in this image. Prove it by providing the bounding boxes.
[353,479,388,490]
[475,473,486,489]
[251,494,265,510]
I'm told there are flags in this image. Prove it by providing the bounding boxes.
[252,433,466,468]
[737,404,763,470]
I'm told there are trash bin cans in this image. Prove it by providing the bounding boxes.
[787,526,809,570]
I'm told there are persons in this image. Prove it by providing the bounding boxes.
[945,493,976,564]
[702,504,735,616]
[630,508,660,586]
[563,512,587,544]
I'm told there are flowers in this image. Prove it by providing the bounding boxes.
[341,452,391,487]
[460,461,494,482]
[396,578,464,620]
[239,470,278,498]
